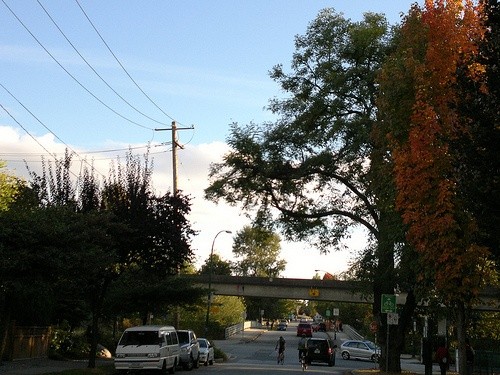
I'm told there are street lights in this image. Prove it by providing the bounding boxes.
[204,230,233,338]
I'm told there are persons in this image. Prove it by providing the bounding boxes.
[297,334,309,365]
[271,323,273,329]
[274,336,286,363]
[266,320,269,329]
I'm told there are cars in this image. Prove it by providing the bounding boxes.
[340,340,382,363]
[277,319,289,332]
[307,338,337,368]
[300,316,308,323]
[196,338,215,366]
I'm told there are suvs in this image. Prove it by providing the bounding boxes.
[176,328,200,371]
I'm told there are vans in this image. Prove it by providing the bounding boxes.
[114,325,180,375]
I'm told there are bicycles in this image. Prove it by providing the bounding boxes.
[275,348,285,365]
[301,351,306,372]
